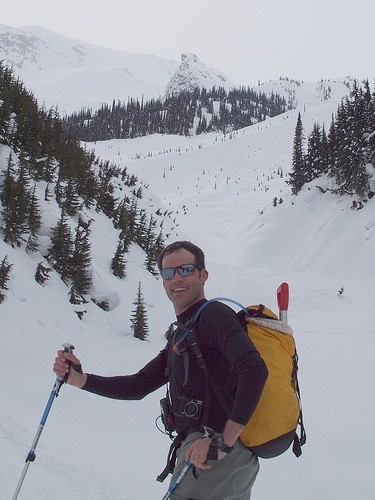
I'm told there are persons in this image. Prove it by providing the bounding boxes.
[53,240,269,500]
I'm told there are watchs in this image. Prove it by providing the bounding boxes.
[217,435,234,453]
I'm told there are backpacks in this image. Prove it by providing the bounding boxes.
[169,297,306,459]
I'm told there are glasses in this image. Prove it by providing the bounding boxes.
[161,263,202,280]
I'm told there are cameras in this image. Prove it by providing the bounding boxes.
[173,395,205,420]
